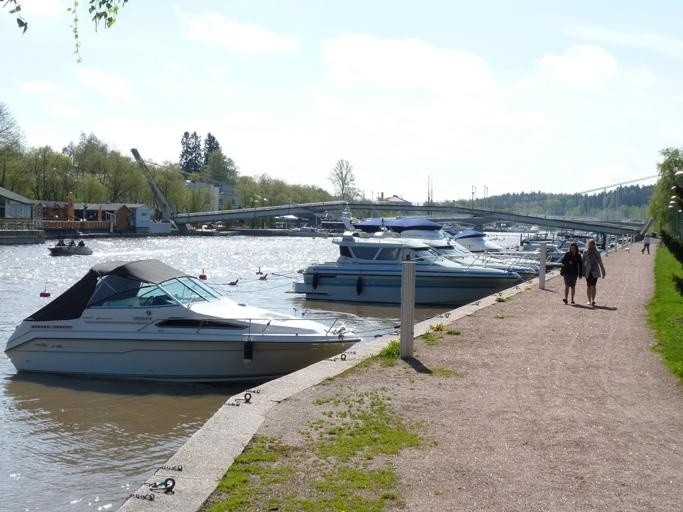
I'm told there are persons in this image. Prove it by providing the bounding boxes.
[641,233,650,254]
[68,239,76,246]
[78,239,85,246]
[56,239,65,246]
[582,238,606,307]
[559,242,583,305]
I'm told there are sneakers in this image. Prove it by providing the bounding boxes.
[588,300,595,305]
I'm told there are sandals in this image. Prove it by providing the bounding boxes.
[562,299,575,304]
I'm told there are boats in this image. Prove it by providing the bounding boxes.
[303,216,617,304]
[48,246,93,256]
[5,259,361,379]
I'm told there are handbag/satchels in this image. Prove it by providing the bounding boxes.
[560,268,566,276]
[592,272,600,278]
